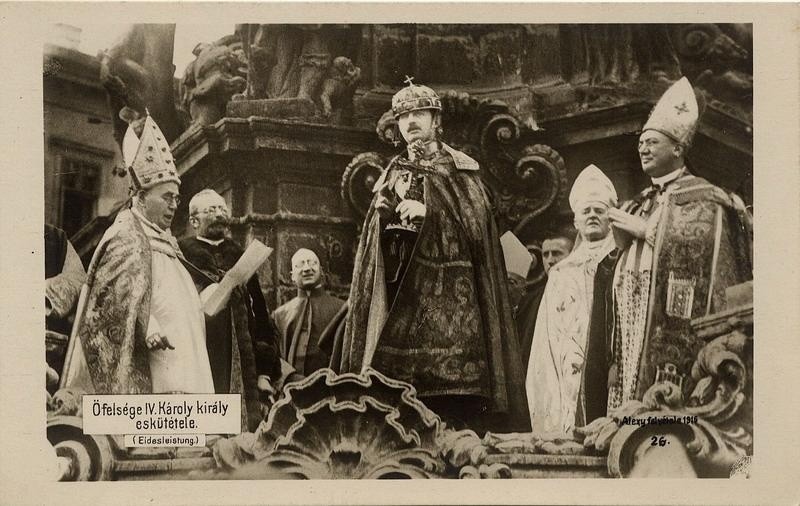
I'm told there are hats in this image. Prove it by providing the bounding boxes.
[569,163,618,213]
[641,75,699,149]
[500,230,534,279]
[121,108,182,198]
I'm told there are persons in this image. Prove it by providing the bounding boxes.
[178,189,283,440]
[317,73,527,432]
[270,247,347,377]
[45,223,87,394]
[59,183,249,396]
[523,232,573,364]
[573,128,753,445]
[506,271,528,342]
[526,201,614,440]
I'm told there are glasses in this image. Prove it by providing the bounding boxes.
[193,209,226,214]
[163,193,183,206]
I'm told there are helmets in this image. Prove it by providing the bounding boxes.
[391,75,442,121]
